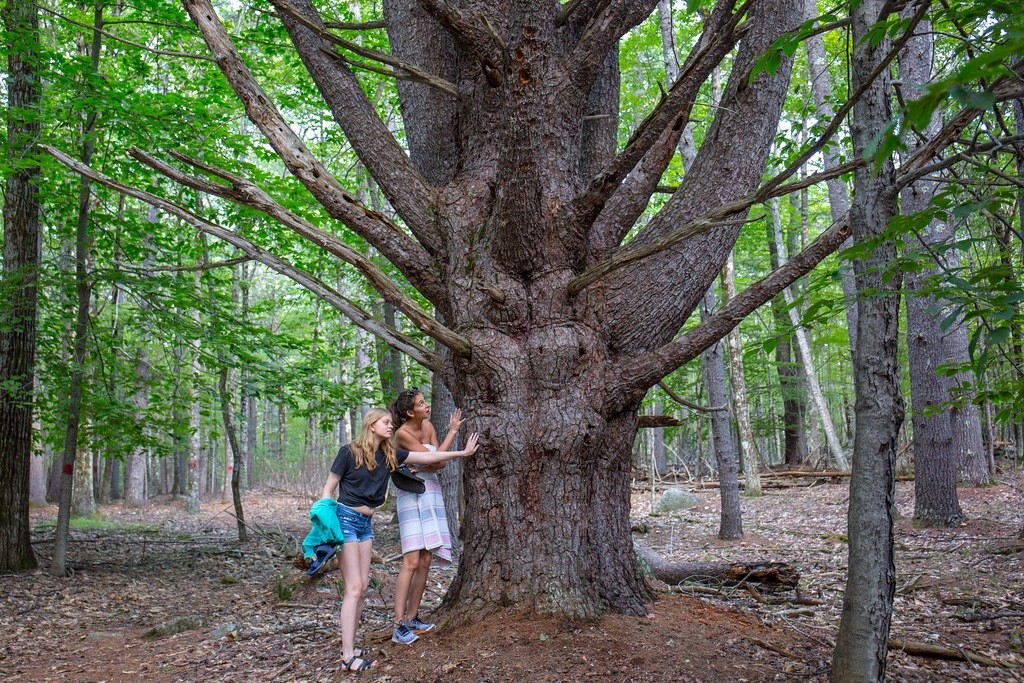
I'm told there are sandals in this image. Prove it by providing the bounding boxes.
[340,646,374,662]
[340,655,379,671]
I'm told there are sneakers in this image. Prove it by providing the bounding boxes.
[404,618,435,632]
[391,619,420,644]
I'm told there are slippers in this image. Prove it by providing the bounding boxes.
[306,543,337,575]
[391,472,425,494]
[396,465,425,484]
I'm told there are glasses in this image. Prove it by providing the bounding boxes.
[404,385,419,408]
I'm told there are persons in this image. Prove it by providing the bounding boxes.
[315,407,480,673]
[391,387,465,644]
